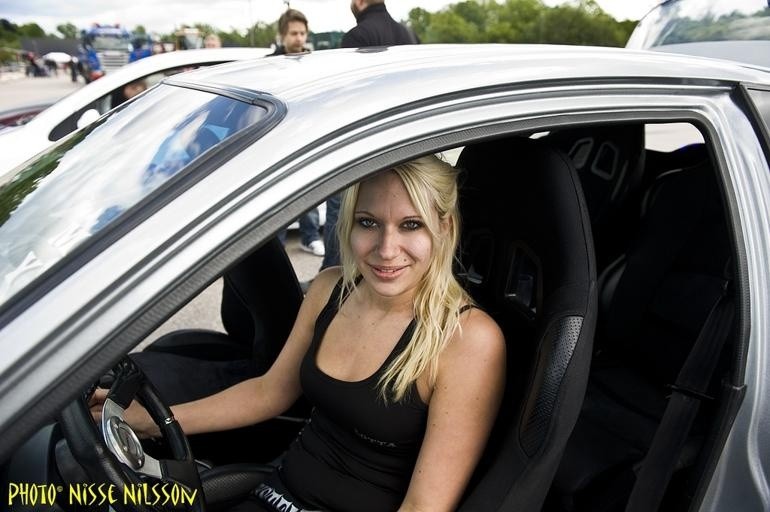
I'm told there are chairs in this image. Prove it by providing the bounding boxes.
[542,168,735,512]
[202,132,597,512]
[129,236,305,478]
[547,123,645,240]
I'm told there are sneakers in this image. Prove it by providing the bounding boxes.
[298,237,326,255]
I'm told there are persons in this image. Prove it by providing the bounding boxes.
[203,34,221,47]
[340,0,421,49]
[111,79,147,110]
[90,154,505,511]
[262,9,315,57]
